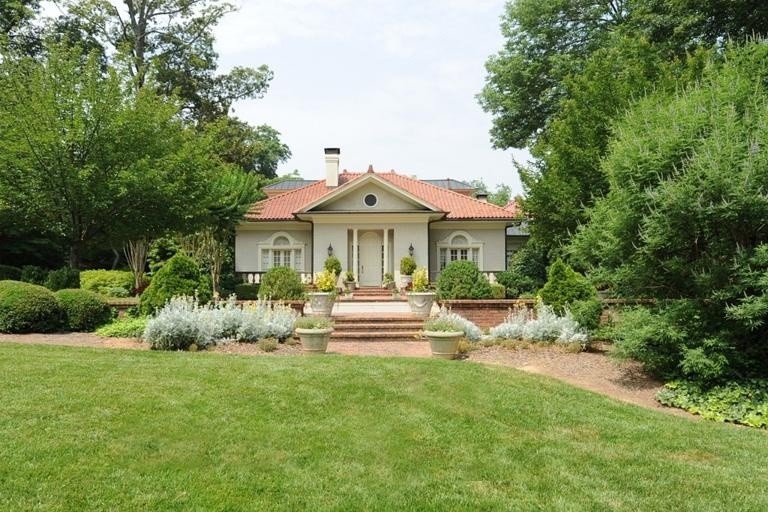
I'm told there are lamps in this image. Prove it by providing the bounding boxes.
[409,243,414,256]
[327,243,334,255]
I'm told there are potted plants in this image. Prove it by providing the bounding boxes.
[399,256,436,312]
[383,273,395,290]
[296,316,336,354]
[308,255,342,315]
[344,272,357,290]
[421,311,465,360]
